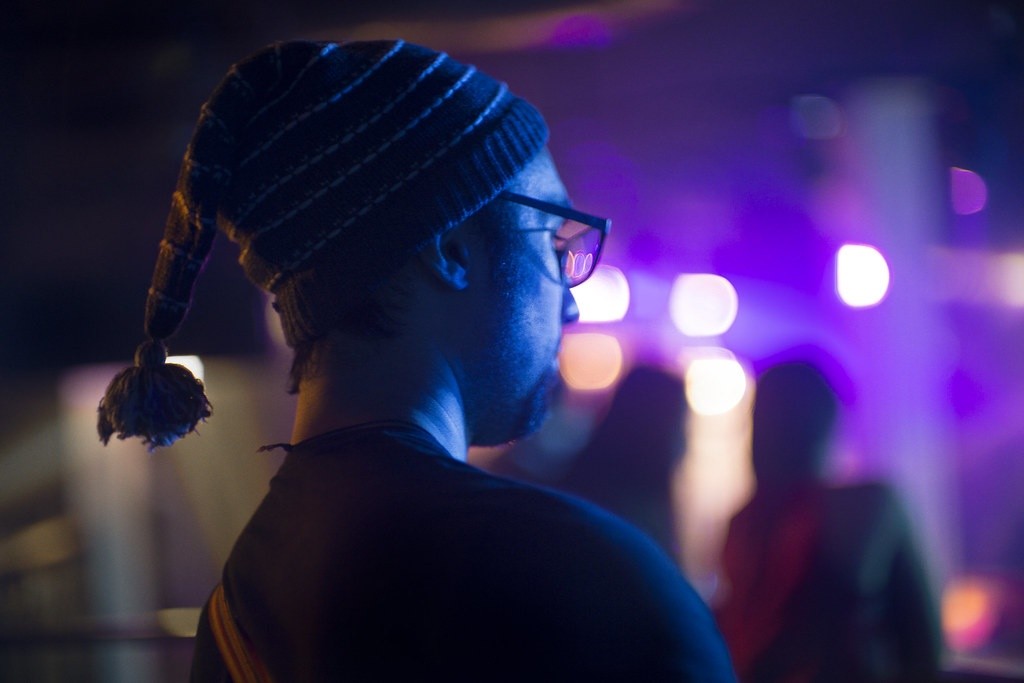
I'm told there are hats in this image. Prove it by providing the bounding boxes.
[97,38,551,451]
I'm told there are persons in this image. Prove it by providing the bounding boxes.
[711,358,948,683]
[190,39,737,683]
[0,457,214,636]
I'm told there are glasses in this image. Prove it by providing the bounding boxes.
[498,190,613,288]
